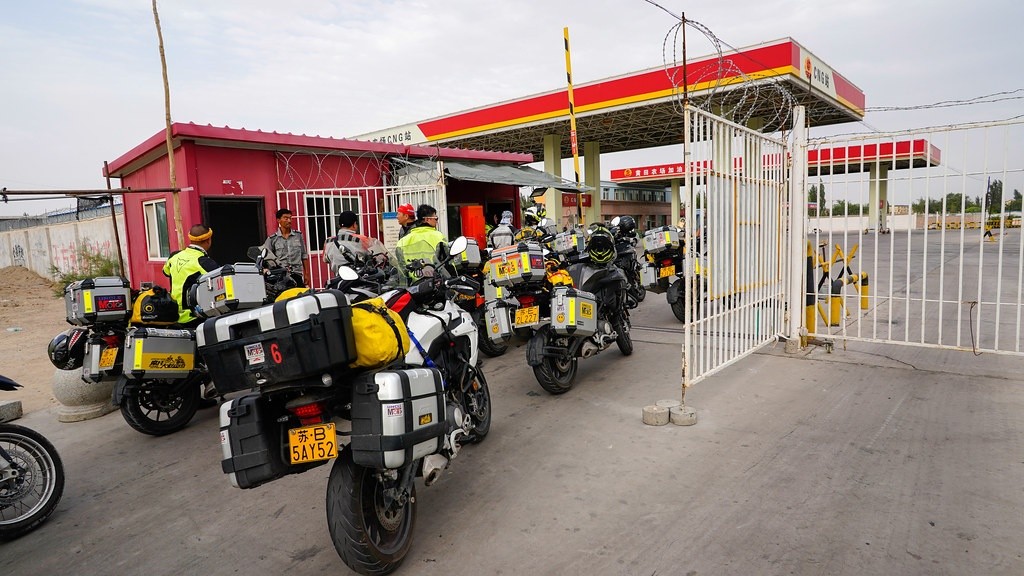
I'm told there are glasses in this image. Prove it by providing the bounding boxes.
[423,216,438,221]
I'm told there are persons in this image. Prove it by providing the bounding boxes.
[264,209,309,288]
[397,205,458,285]
[396,203,416,240]
[484,210,518,249]
[323,211,369,277]
[162,224,221,328]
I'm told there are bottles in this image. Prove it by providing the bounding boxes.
[6,328,23,332]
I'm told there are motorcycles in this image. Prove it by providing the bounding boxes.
[65,214,740,437]
[0,375,65,542]
[193,234,493,576]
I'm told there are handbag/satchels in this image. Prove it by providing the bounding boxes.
[132,286,179,324]
[277,283,409,368]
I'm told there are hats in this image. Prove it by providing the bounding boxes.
[339,210,359,228]
[397,203,414,216]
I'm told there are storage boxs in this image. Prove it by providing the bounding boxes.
[194,284,359,392]
[65,273,134,326]
[642,223,679,256]
[353,365,446,469]
[489,241,546,289]
[482,298,516,345]
[192,259,265,317]
[121,325,195,381]
[556,227,583,255]
[681,258,707,281]
[635,264,671,293]
[448,235,480,267]
[82,335,116,383]
[552,285,599,337]
[217,383,311,489]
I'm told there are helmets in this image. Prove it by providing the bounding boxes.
[47,328,87,371]
[587,227,616,264]
[619,216,635,233]
[525,207,542,223]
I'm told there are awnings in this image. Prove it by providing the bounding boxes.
[392,156,592,194]
